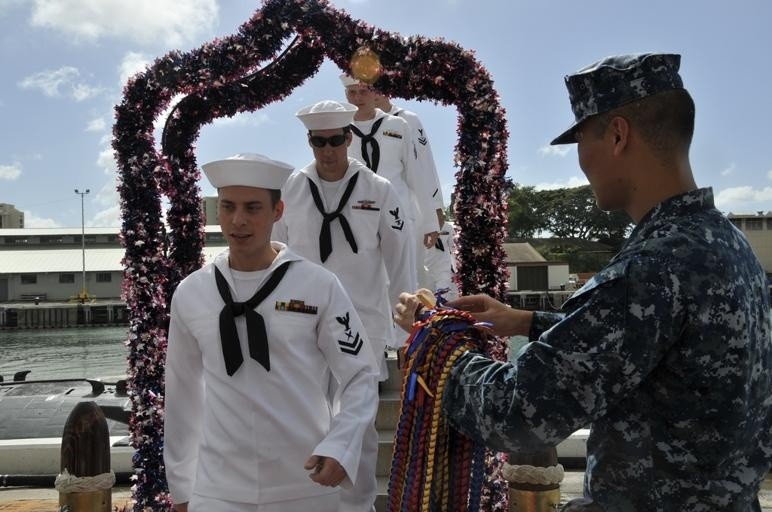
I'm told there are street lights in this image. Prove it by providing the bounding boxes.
[74,186,93,305]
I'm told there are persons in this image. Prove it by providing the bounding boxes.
[161,151,381,511]
[422,218,461,302]
[374,84,446,231]
[390,48,772,512]
[337,68,440,352]
[270,97,415,408]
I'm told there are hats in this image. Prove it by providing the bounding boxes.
[296,100,360,130]
[339,72,361,86]
[548,49,682,142]
[201,152,294,193]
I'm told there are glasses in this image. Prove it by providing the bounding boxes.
[309,135,347,148]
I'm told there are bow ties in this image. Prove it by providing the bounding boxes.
[211,261,294,376]
[305,169,362,264]
[349,117,385,174]
